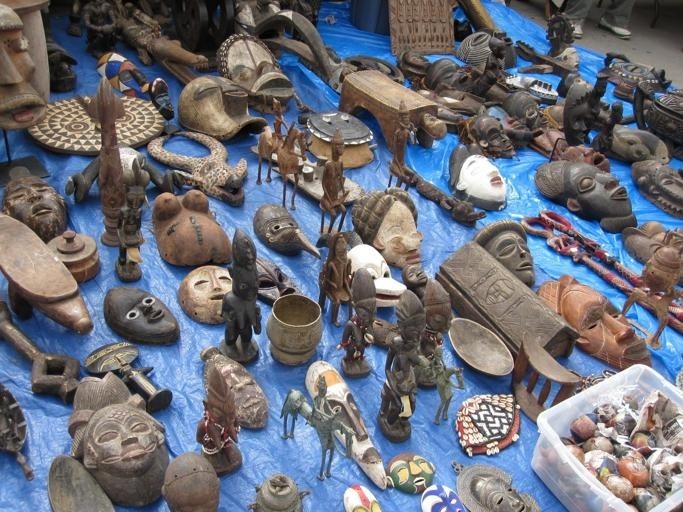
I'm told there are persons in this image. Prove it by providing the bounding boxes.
[551,0,637,39]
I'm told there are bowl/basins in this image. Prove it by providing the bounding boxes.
[448,317,514,376]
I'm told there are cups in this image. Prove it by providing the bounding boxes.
[266,295,323,365]
